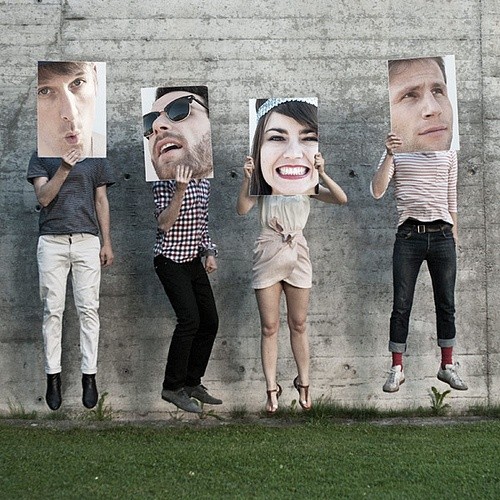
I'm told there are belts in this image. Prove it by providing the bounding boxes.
[403,224,447,234]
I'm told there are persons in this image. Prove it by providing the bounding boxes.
[249,99,319,196]
[143,85,213,180]
[237,151,347,414]
[387,57,454,153]
[370,132,469,393]
[37,60,99,157]
[153,166,223,414]
[26,148,116,411]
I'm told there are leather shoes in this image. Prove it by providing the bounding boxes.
[81,373,98,409]
[45,373,62,409]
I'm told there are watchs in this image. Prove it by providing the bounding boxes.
[206,249,219,258]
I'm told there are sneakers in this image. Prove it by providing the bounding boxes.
[161,384,223,413]
[383,365,405,393]
[437,362,468,390]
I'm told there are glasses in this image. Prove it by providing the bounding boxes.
[143,94,209,140]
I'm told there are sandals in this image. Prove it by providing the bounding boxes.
[265,382,282,414]
[294,376,312,411]
